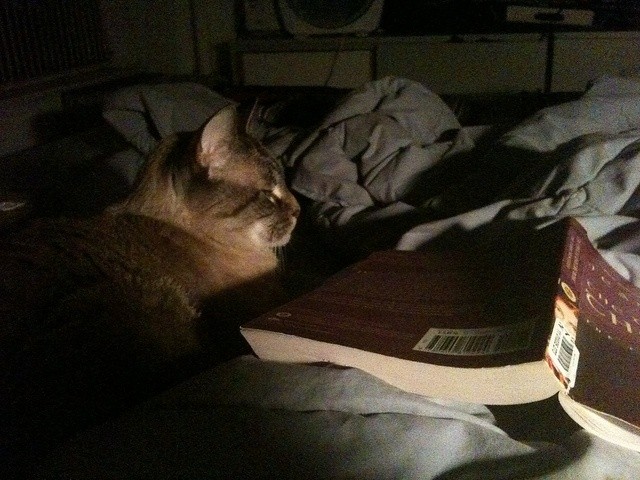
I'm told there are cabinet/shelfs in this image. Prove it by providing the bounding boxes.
[226,32,639,88]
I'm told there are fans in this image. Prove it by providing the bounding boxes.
[278,0,383,37]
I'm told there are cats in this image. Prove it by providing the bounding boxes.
[0,99,301,432]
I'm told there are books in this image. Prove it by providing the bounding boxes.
[239,212,640,453]
